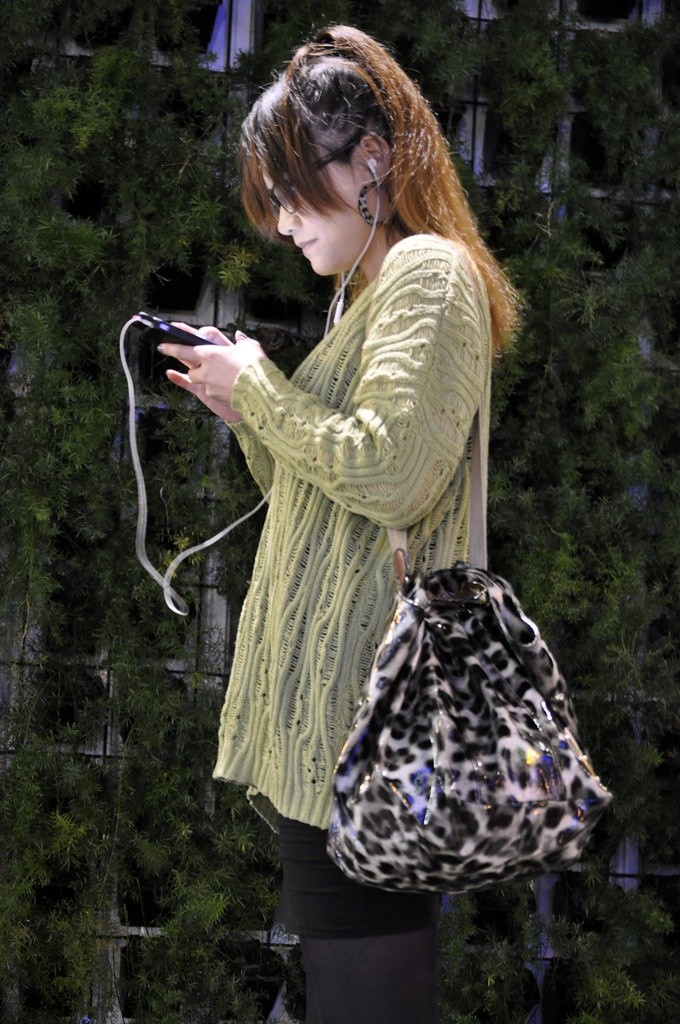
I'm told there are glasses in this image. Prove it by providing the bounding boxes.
[267,136,360,215]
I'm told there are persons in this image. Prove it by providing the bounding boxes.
[151,21,527,1024]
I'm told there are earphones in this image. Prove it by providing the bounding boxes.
[367,159,378,173]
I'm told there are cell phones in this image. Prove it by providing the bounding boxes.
[142,321,217,346]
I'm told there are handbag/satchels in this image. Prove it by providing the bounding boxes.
[329,565,612,896]
